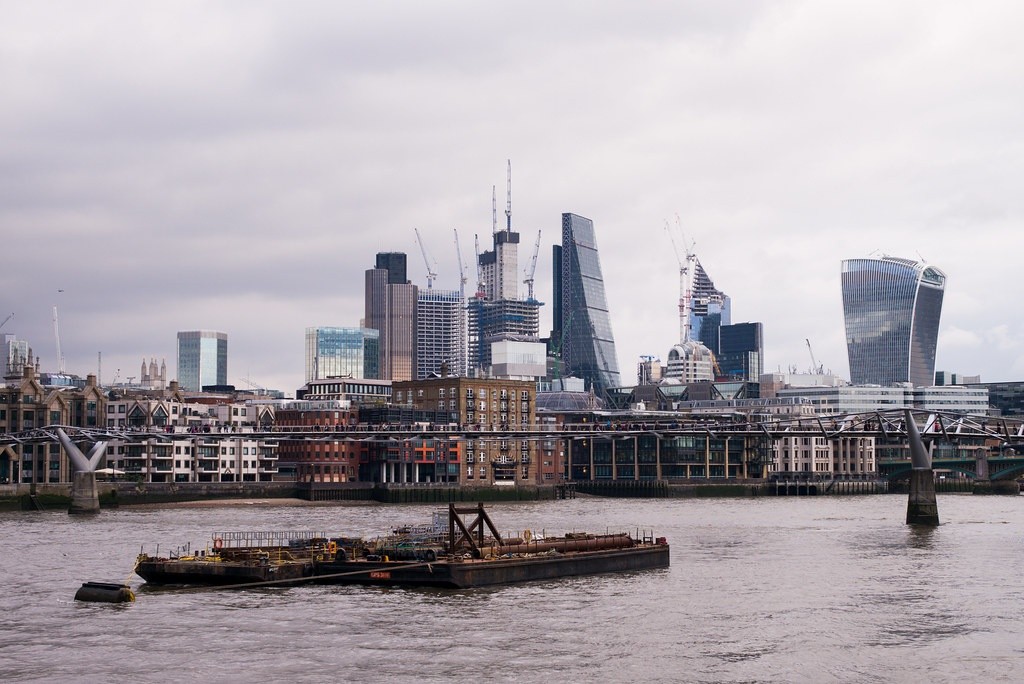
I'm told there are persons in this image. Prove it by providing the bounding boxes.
[475,422,509,431]
[166,424,175,433]
[315,422,344,432]
[187,422,213,434]
[981,421,1018,435]
[830,418,907,431]
[217,421,237,433]
[681,418,719,432]
[743,420,751,431]
[593,418,662,431]
[97,423,148,434]
[407,423,434,431]
[377,421,393,431]
[253,423,258,432]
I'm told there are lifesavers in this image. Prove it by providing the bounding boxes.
[329,542,336,551]
[214,538,222,549]
[425,548,436,561]
[523,529,532,540]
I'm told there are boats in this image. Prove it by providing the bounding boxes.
[135,501,669,590]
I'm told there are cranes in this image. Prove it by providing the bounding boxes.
[53,306,65,374]
[664,211,697,343]
[414,158,542,365]
[550,309,576,377]
[0,312,15,329]
[805,339,824,374]
[112,368,120,386]
[97,350,103,388]
